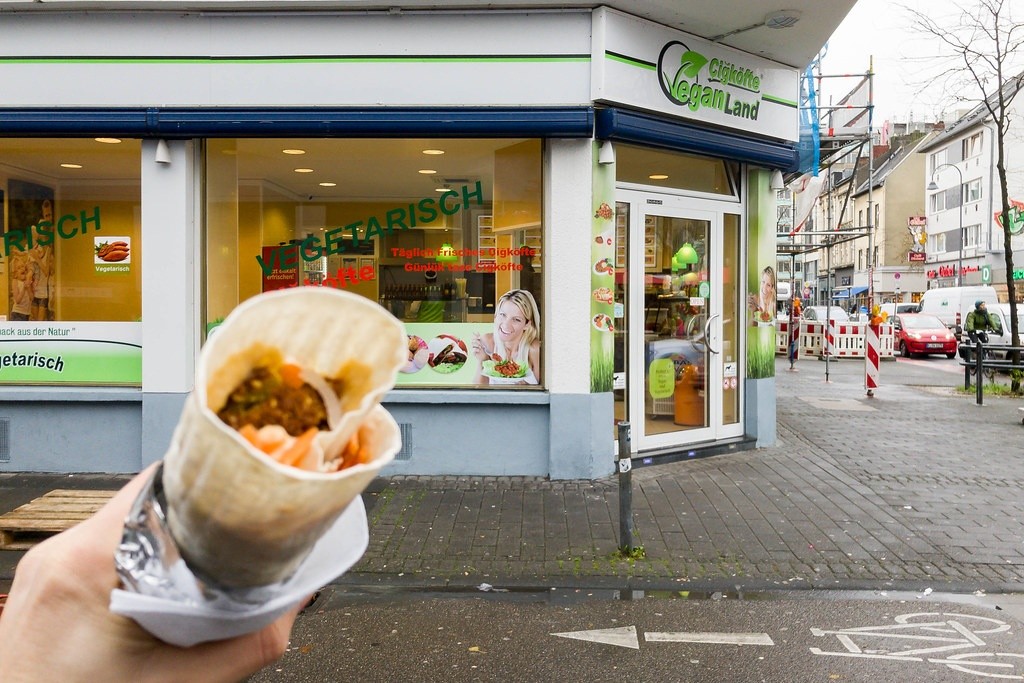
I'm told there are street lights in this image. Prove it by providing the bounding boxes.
[926,162,964,287]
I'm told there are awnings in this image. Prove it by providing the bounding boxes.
[832,287,868,298]
[832,286,852,292]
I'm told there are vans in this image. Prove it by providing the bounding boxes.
[913,287,998,341]
[874,303,920,314]
[957,305,1024,372]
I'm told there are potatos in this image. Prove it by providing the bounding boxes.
[97,241,129,260]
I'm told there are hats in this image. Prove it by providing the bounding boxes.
[975,299,985,309]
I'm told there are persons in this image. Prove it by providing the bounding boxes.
[967,301,1003,360]
[473,289,540,385]
[411,268,446,322]
[748,266,776,326]
[10,198,56,320]
[520,245,537,295]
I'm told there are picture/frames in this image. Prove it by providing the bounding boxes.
[326,256,376,280]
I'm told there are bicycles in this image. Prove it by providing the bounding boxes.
[968,329,1003,377]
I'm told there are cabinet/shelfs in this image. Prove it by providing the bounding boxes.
[478,214,657,267]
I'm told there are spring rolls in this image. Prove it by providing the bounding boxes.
[161,286,409,590]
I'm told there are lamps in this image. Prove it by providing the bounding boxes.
[671,219,698,272]
[764,10,801,30]
[436,184,458,261]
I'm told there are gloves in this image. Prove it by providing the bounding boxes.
[996,330,1003,336]
[968,331,978,343]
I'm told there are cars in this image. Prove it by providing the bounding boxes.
[849,314,873,324]
[803,306,850,324]
[886,313,957,359]
[647,318,732,376]
